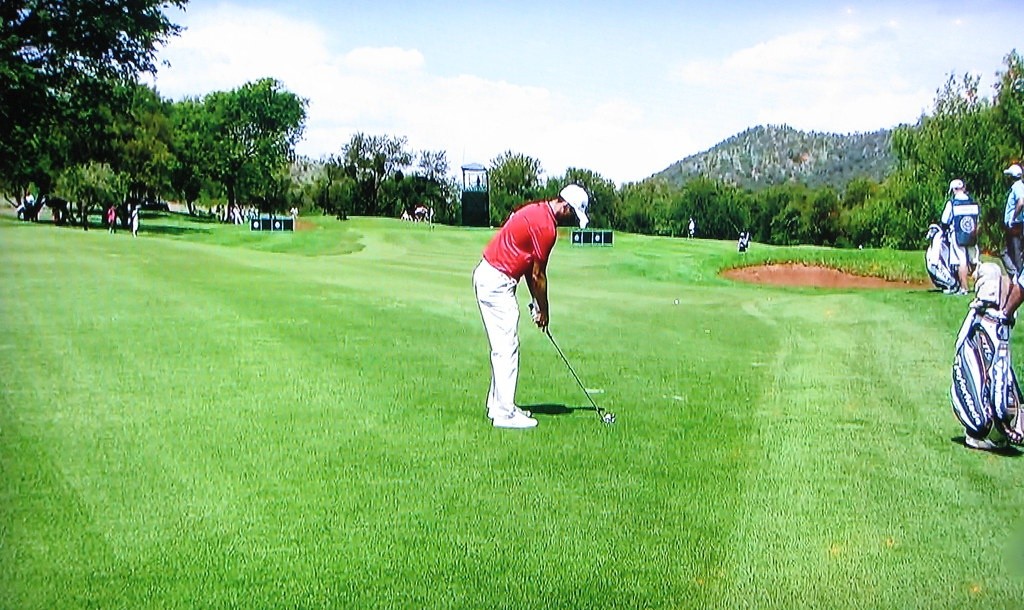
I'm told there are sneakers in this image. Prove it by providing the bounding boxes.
[493,411,538,428]
[487,407,532,419]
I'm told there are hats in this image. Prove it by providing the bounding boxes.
[947,179,963,194]
[1004,164,1022,177]
[560,184,589,223]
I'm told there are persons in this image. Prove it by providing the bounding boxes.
[289,206,298,218]
[940,179,981,295]
[472,185,589,429]
[26,192,34,203]
[216,202,255,225]
[107,203,141,238]
[1000,164,1024,279]
[400,204,429,223]
[687,217,695,239]
[1002,268,1024,330]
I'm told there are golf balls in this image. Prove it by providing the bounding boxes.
[674,300,678,304]
[604,413,614,422]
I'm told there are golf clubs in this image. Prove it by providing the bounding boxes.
[528,303,611,424]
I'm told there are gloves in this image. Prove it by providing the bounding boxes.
[528,303,540,327]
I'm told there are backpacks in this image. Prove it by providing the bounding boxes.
[948,194,979,246]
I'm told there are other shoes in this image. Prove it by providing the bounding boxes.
[956,287,967,295]
